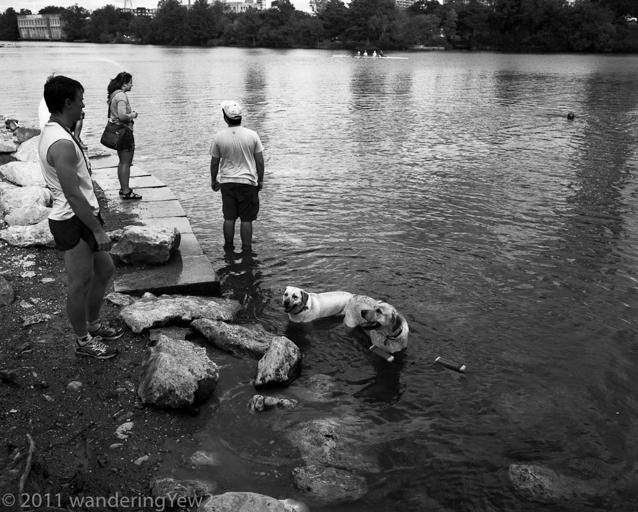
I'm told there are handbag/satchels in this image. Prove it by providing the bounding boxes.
[99,120,128,150]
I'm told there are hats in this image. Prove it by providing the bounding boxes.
[220,100,244,120]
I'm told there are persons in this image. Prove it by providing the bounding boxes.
[209,100,265,254]
[39,74,128,362]
[106,70,143,201]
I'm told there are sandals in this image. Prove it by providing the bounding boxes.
[118,187,142,200]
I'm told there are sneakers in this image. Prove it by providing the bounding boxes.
[74,337,119,360]
[87,322,125,341]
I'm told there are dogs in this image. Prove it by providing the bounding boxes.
[337,294,409,353]
[283,286,353,322]
